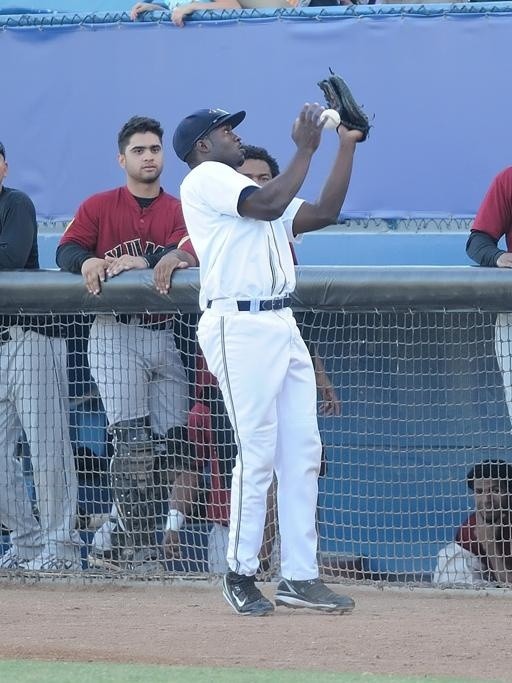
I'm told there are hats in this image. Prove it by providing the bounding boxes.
[173,106,246,159]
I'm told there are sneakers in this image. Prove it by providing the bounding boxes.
[222,572,273,615]
[0,548,83,574]
[89,548,163,577]
[275,576,355,614]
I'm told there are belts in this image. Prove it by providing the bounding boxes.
[114,313,174,330]
[207,296,293,312]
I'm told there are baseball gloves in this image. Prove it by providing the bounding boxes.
[317,66,370,142]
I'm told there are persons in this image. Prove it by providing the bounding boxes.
[129,0,242,27]
[173,100,364,615]
[160,397,277,581]
[57,116,191,575]
[153,142,344,420]
[0,136,83,574]
[464,165,511,427]
[433,457,511,588]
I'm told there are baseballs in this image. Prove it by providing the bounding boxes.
[319,108,341,131]
